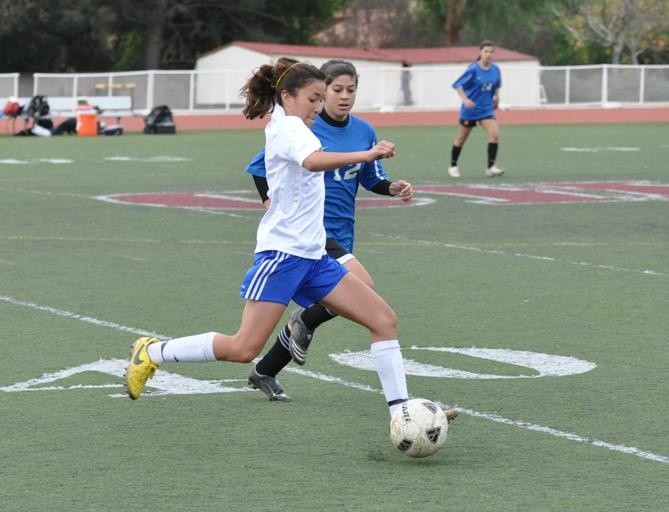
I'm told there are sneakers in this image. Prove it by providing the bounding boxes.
[448,166,461,178]
[444,409,458,423]
[125,336,159,399]
[248,363,290,401]
[289,307,314,365]
[486,165,505,177]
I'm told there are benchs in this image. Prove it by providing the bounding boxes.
[8,94,136,134]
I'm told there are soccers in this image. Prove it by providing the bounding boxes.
[390,399,449,457]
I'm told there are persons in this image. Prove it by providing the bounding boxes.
[123,58,462,423]
[245,56,414,403]
[446,39,511,183]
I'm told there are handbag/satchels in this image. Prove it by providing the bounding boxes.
[145,106,173,130]
[27,95,48,117]
